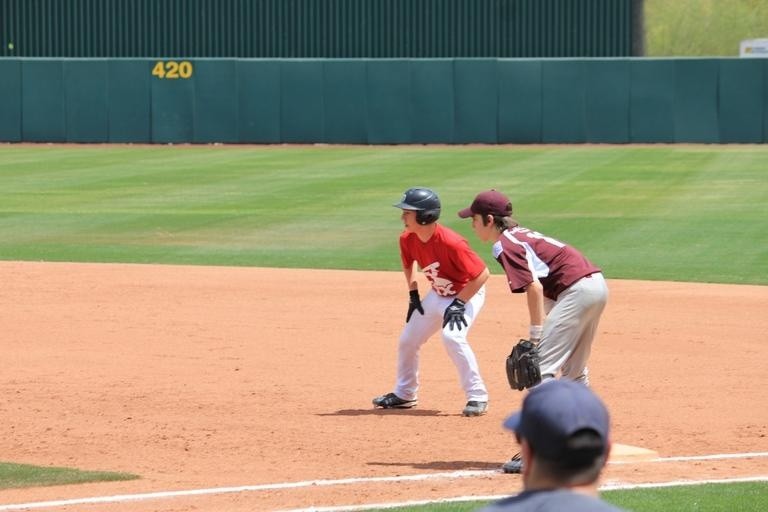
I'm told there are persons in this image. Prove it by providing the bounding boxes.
[458,190,608,472]
[474,378,629,512]
[373,189,491,415]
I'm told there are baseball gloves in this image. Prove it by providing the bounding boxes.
[504,338,542,391]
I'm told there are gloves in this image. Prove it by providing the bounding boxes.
[405,289,425,322]
[441,298,468,331]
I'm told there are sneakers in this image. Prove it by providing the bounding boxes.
[502,452,524,473]
[462,401,488,417]
[373,393,418,407]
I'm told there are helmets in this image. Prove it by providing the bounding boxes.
[392,187,441,225]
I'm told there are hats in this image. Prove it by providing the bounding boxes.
[458,189,513,218]
[502,378,611,460]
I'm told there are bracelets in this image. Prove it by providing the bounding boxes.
[529,325,542,339]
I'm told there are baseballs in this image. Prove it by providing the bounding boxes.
[7,43,15,50]
